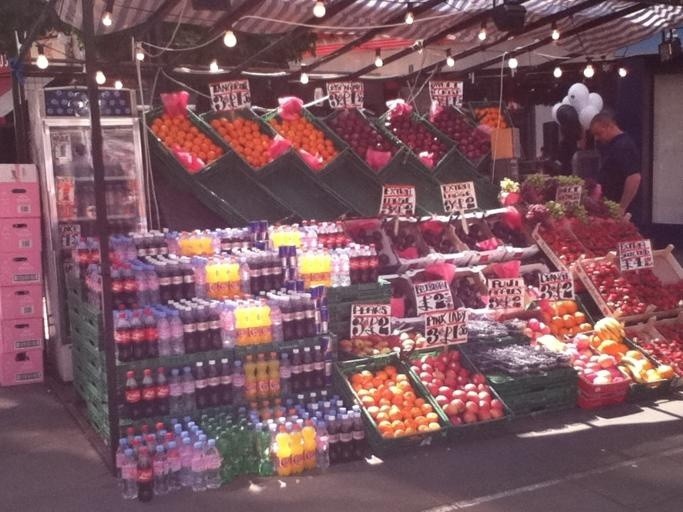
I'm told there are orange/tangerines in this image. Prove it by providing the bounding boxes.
[351,373,363,384]
[411,387,416,395]
[404,418,417,428]
[374,392,382,401]
[392,389,405,397]
[351,383,362,392]
[421,403,433,415]
[401,407,410,417]
[375,412,390,423]
[394,429,405,437]
[396,374,410,382]
[392,420,405,431]
[362,395,376,408]
[379,420,392,432]
[376,371,388,381]
[380,404,391,412]
[367,406,380,418]
[417,424,429,431]
[378,397,391,407]
[382,388,393,401]
[361,379,369,385]
[411,407,423,418]
[399,380,411,392]
[371,377,384,387]
[403,391,416,403]
[388,386,399,392]
[369,388,378,397]
[384,431,394,439]
[426,412,439,423]
[414,398,425,407]
[403,400,415,407]
[377,384,386,393]
[405,427,416,436]
[403,414,412,420]
[364,381,374,390]
[415,416,428,428]
[357,389,371,398]
[384,379,396,388]
[391,395,403,407]
[384,366,398,378]
[389,411,403,421]
[390,404,401,413]
[361,370,373,382]
[429,422,441,430]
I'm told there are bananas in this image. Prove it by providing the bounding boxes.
[624,362,641,383]
[618,357,642,373]
[594,317,626,343]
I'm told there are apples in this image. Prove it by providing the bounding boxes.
[573,334,590,350]
[351,339,364,349]
[421,353,432,365]
[421,363,433,374]
[456,384,464,391]
[477,384,489,392]
[448,361,461,374]
[439,352,450,366]
[420,372,433,383]
[490,399,503,410]
[452,389,468,402]
[592,363,602,371]
[574,366,584,372]
[612,376,624,383]
[528,318,539,331]
[415,339,423,348]
[351,347,362,353]
[579,349,592,358]
[378,341,389,347]
[465,400,479,413]
[363,347,374,355]
[432,378,443,387]
[529,339,537,345]
[593,376,608,385]
[478,408,491,420]
[445,369,458,379]
[465,383,477,392]
[533,331,543,339]
[414,333,423,340]
[585,374,599,383]
[611,369,622,378]
[546,325,551,334]
[432,367,445,384]
[435,395,450,406]
[386,335,402,349]
[418,337,426,343]
[539,322,547,334]
[438,386,453,402]
[373,344,383,351]
[363,340,374,346]
[579,354,589,364]
[368,335,383,344]
[489,408,505,418]
[433,361,445,372]
[411,359,422,368]
[478,390,492,403]
[566,348,580,359]
[584,368,595,375]
[408,333,415,339]
[444,377,457,390]
[471,373,486,386]
[608,365,617,372]
[442,403,458,417]
[463,411,477,423]
[427,382,439,397]
[456,375,469,386]
[589,355,600,362]
[466,391,478,402]
[458,367,468,378]
[381,347,391,354]
[448,350,461,362]
[523,328,533,338]
[585,362,597,369]
[573,360,586,369]
[595,369,612,377]
[339,338,352,353]
[450,399,465,414]
[451,413,465,425]
[410,365,420,375]
[597,354,613,369]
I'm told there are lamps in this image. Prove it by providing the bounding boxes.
[508,52,518,69]
[551,23,560,40]
[136,47,144,61]
[102,7,113,27]
[224,25,237,48]
[618,61,626,77]
[553,61,562,78]
[479,22,487,40]
[36,46,48,69]
[583,60,594,78]
[301,65,309,85]
[210,54,219,72]
[446,48,454,67]
[405,6,413,25]
[115,75,122,89]
[375,48,383,67]
[95,67,106,85]
[313,0,325,18]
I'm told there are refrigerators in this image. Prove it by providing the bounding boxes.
[28,86,149,385]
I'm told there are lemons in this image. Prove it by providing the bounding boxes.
[618,344,629,353]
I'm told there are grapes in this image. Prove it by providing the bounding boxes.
[525,204,552,223]
[545,201,565,221]
[604,200,622,220]
[500,177,521,193]
[572,204,589,225]
[546,175,586,194]
[583,194,610,214]
[526,172,546,195]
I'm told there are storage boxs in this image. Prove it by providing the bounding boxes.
[607,250,681,321]
[531,222,597,280]
[0,286,43,319]
[454,268,489,316]
[337,355,453,457]
[424,104,492,170]
[474,264,531,314]
[573,250,656,324]
[0,164,41,218]
[0,251,42,286]
[341,218,401,274]
[619,321,683,390]
[448,212,507,267]
[0,318,43,350]
[408,269,465,318]
[484,205,539,262]
[140,103,231,182]
[198,106,294,177]
[376,110,459,175]
[561,339,631,409]
[323,110,406,177]
[0,349,44,385]
[320,151,384,219]
[404,344,515,435]
[519,263,550,301]
[259,161,353,223]
[377,273,426,326]
[260,106,350,174]
[0,218,42,251]
[381,217,439,274]
[379,160,446,215]
[415,216,472,267]
[434,150,501,211]
[468,99,513,128]
[191,162,295,228]
[463,337,580,418]
[582,329,673,402]
[492,127,522,159]
[651,244,683,316]
[646,316,683,343]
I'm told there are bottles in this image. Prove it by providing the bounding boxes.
[179,439,190,489]
[214,301,235,348]
[244,355,257,400]
[169,369,183,413]
[370,244,379,281]
[221,247,278,258]
[158,314,170,355]
[322,308,328,334]
[328,417,339,464]
[304,421,317,470]
[256,353,269,398]
[291,350,303,391]
[350,247,360,287]
[318,422,328,467]
[131,312,146,359]
[153,445,167,495]
[45,80,130,117]
[154,369,169,414]
[252,259,262,293]
[288,281,295,290]
[191,442,204,493]
[141,370,155,416]
[90,238,100,263]
[117,314,131,361]
[314,345,325,387]
[305,348,314,389]
[258,424,273,476]
[236,289,311,303]
[260,299,270,342]
[232,360,244,403]
[320,287,328,306]
[323,337,331,358]
[167,299,228,318]
[146,310,159,356]
[291,424,306,473]
[261,221,268,241]
[84,267,101,310]
[205,361,220,405]
[361,245,369,283]
[145,256,185,267]
[125,372,141,420]
[183,368,195,413]
[353,413,365,459]
[269,220,346,254]
[250,221,261,244]
[190,406,256,482]
[111,267,123,307]
[117,439,129,480]
[298,245,351,287]
[243,228,250,249]
[182,307,196,354]
[124,264,137,307]
[326,361,333,384]
[139,447,153,502]
[119,297,218,314]
[182,259,195,299]
[297,280,303,291]
[171,262,183,298]
[276,426,293,476]
[148,236,157,258]
[208,304,223,348]
[231,230,242,253]
[341,415,354,464]
[110,232,148,259]
[170,310,185,353]
[312,286,318,307]
[281,355,291,390]
[293,299,305,337]
[280,246,288,285]
[272,253,282,292]
[160,264,172,303]
[222,359,232,404]
[194,258,238,298]
[270,302,283,342]
[204,439,222,489]
[290,246,297,283]
[269,352,280,395]
[248,301,259,344]
[78,236,88,266]
[137,266,147,302]
[166,230,221,257]
[127,415,208,442]
[221,228,231,253]
[159,235,166,259]
[250,391,360,431]
[240,259,251,292]
[283,297,293,340]
[196,306,210,350]
[167,443,179,489]
[236,302,246,345]
[264,256,271,290]
[315,308,321,334]
[148,267,159,303]
[306,295,314,337]
[196,363,205,408]
[121,449,138,497]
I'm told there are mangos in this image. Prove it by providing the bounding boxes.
[639,359,656,369]
[626,350,642,360]
[646,369,661,381]
[592,335,600,347]
[615,352,624,361]
[657,365,673,379]
[596,340,618,354]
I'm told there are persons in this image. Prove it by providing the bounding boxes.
[589,112,642,225]
